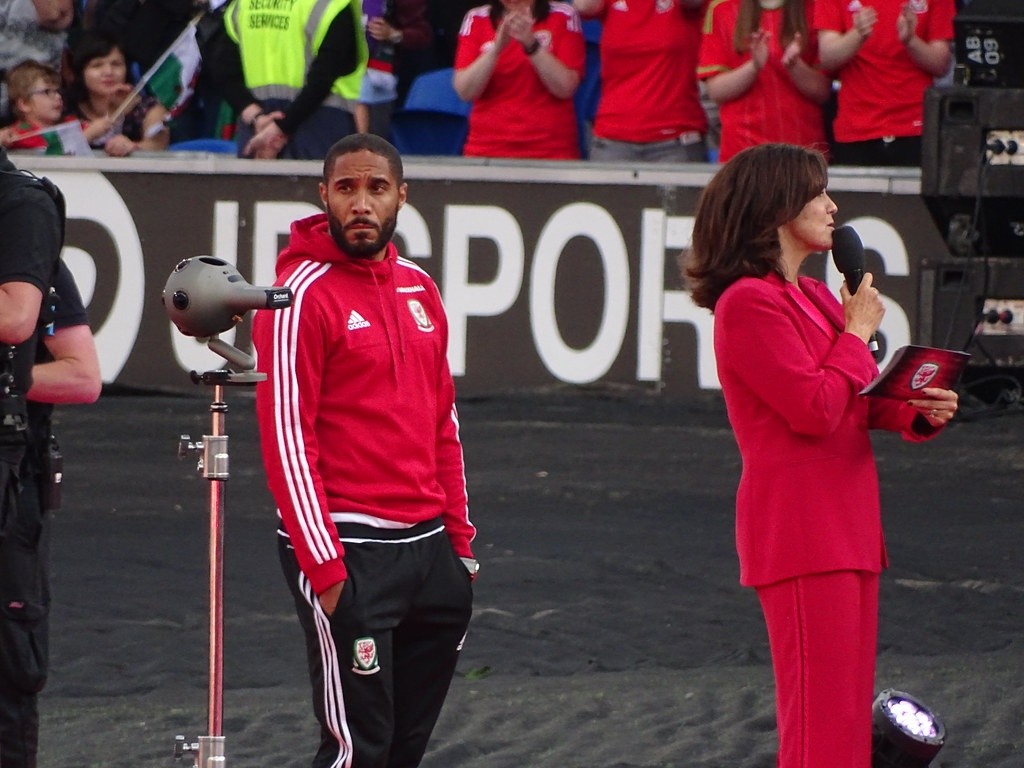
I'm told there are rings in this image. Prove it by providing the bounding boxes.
[931,409,938,418]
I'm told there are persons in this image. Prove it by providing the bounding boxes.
[252,133,480,768]
[0,142,102,766]
[685,142,959,766]
[0,0,958,168]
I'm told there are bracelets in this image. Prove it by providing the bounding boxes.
[525,38,538,51]
[526,42,539,54]
[248,110,268,126]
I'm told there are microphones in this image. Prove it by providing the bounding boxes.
[830,225,879,358]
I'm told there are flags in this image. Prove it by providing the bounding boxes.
[145,25,203,115]
[42,124,94,158]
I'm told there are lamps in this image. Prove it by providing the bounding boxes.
[871,688,945,768]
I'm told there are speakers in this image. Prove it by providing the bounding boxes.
[917,85,1024,256]
[918,255,1024,407]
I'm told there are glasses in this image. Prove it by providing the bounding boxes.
[21,85,62,96]
